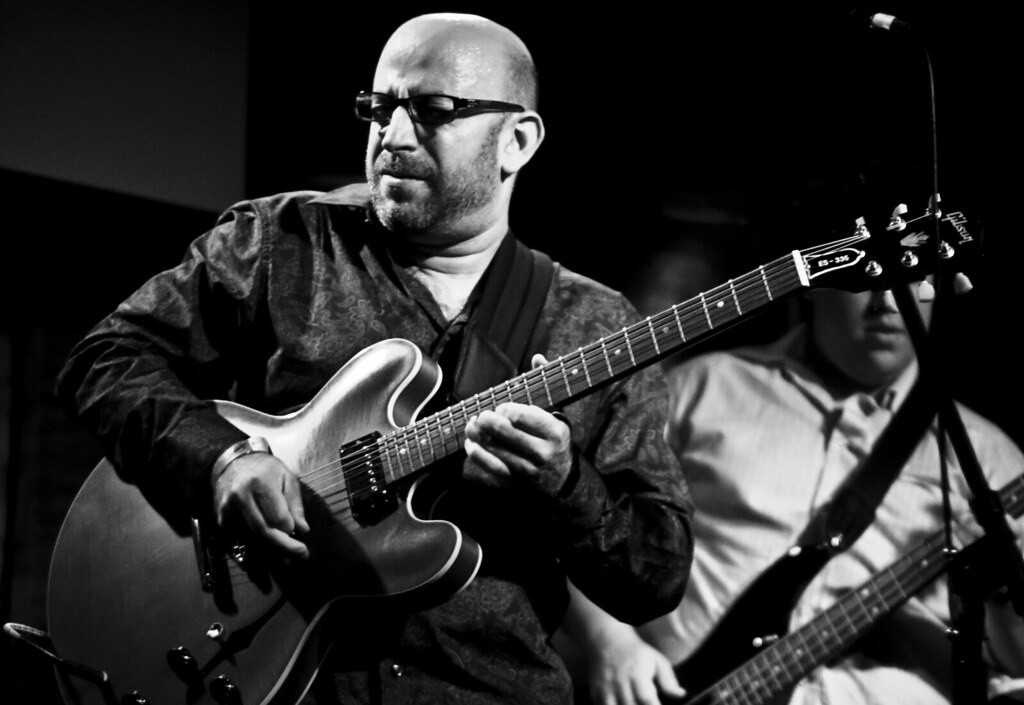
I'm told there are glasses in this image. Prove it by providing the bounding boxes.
[353,90,524,125]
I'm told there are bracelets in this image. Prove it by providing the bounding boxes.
[209,438,272,488]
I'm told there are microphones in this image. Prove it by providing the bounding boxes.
[848,9,911,33]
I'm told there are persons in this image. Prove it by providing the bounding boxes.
[45,13,695,705]
[560,159,1024,705]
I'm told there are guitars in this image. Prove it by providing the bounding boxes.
[40,186,980,705]
[687,471,1024,704]
